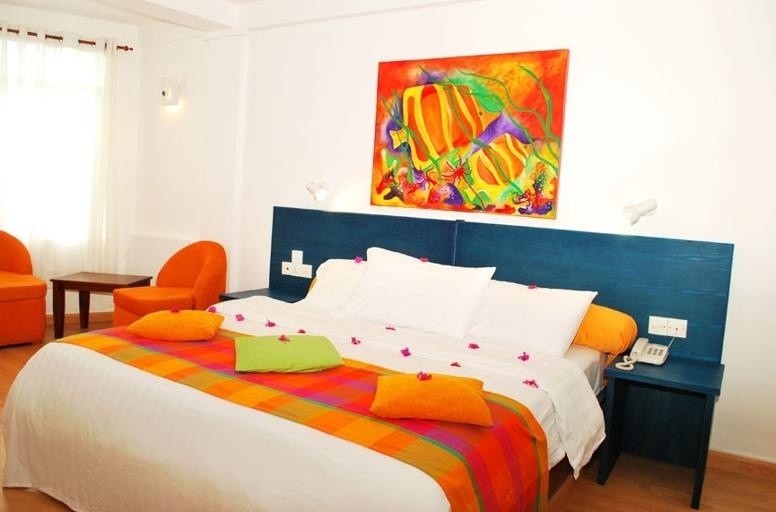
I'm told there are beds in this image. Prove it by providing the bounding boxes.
[14,202,735,512]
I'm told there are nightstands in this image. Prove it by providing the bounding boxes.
[596,348,728,511]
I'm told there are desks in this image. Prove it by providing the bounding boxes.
[51,270,153,340]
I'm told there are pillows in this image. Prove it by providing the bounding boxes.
[369,368,497,431]
[124,305,341,377]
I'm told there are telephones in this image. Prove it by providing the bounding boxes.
[630,338,670,366]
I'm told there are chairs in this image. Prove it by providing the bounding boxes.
[0,230,47,348]
[111,239,228,326]
